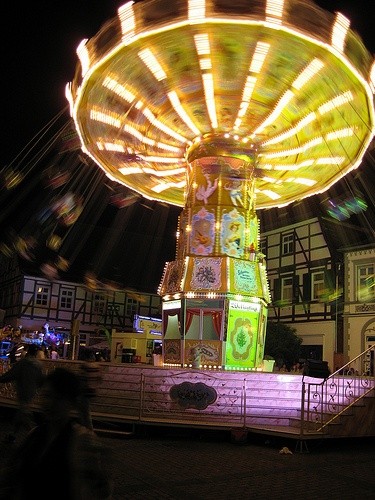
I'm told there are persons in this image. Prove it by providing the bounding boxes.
[0,326,69,374]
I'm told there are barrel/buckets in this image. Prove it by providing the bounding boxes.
[121,348,136,363]
[132,355,141,363]
[261,359,275,373]
